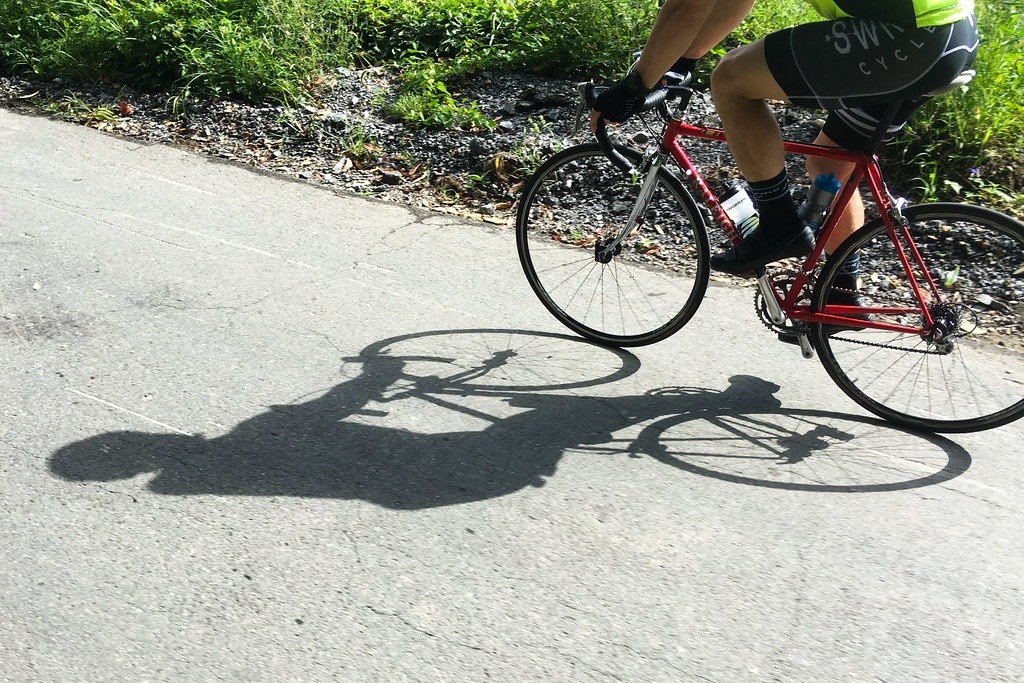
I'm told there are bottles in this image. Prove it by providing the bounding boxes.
[718,182,760,239]
[797,172,842,240]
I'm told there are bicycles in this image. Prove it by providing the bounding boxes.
[515,50,1024,434]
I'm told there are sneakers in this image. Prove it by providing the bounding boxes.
[778,296,872,345]
[711,221,818,272]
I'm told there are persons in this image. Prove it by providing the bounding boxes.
[589,0,980,348]
